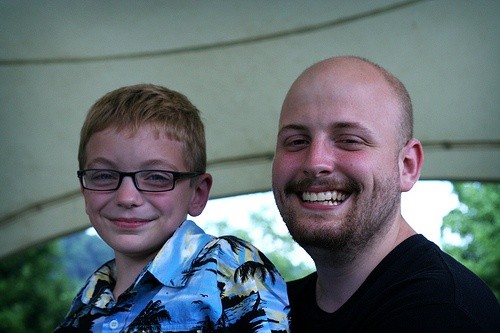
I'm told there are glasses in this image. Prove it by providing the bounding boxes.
[76,169,196,192]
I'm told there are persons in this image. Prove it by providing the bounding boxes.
[47,83,293,333]
[270,54,500,333]
